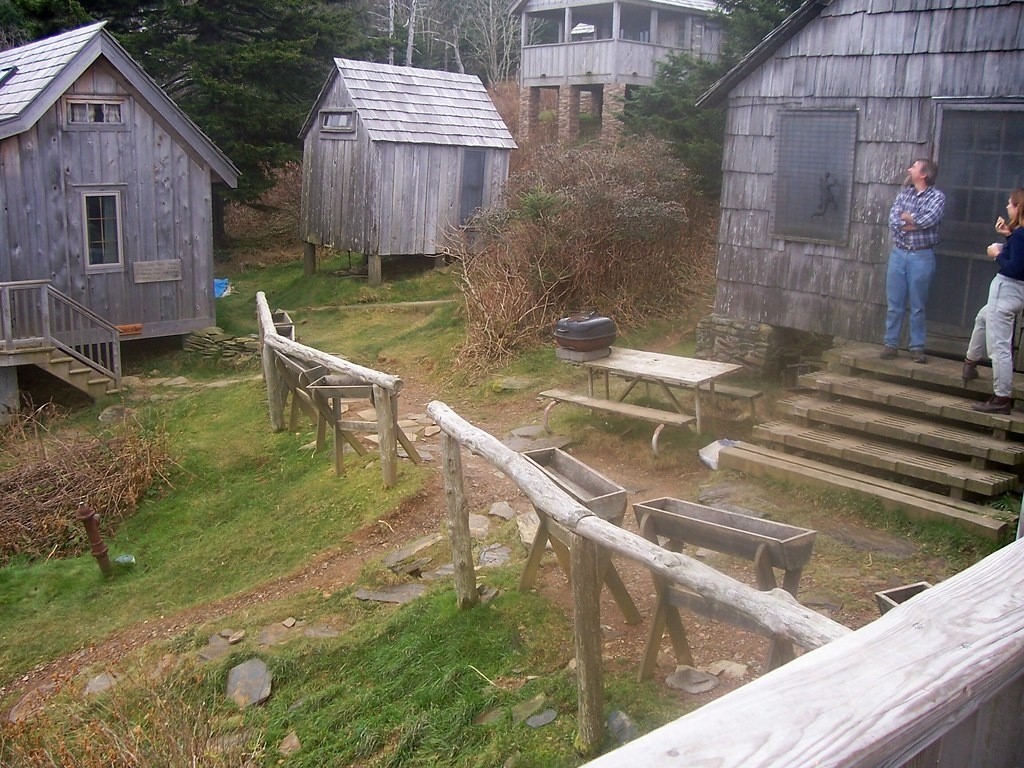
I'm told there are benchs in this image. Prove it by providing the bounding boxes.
[538,387,697,459]
[610,371,763,422]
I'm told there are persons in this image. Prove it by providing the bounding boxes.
[879,158,946,363]
[962,187,1024,415]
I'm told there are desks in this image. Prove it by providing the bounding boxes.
[559,346,744,436]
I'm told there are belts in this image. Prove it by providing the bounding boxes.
[896,241,932,251]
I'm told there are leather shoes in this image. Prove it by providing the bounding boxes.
[963,358,979,379]
[973,392,1015,415]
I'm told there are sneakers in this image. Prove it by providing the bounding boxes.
[879,346,897,359]
[912,350,927,363]
